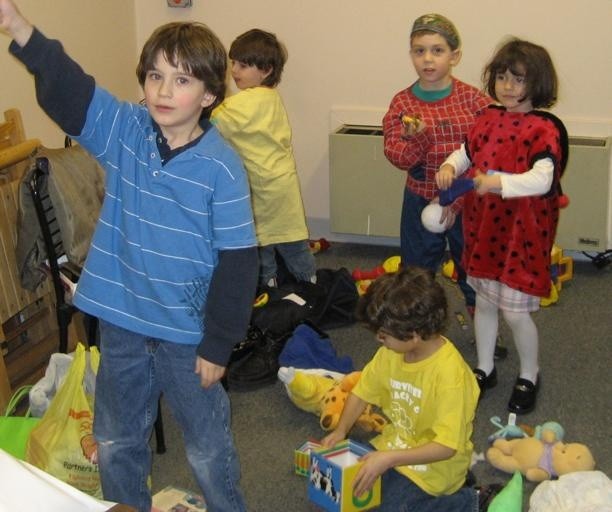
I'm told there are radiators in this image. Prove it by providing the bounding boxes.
[325,106,611,269]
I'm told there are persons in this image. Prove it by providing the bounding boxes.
[381,12,509,356]
[2,2,261,512]
[430,37,570,410]
[318,264,486,512]
[205,30,319,292]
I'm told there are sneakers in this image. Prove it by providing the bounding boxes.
[495,345,508,361]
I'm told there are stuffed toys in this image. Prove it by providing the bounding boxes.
[277,323,388,433]
[489,414,597,483]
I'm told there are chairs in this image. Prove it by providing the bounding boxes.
[27,140,229,455]
[0,108,90,413]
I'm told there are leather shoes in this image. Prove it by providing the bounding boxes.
[507,372,540,415]
[473,366,499,399]
